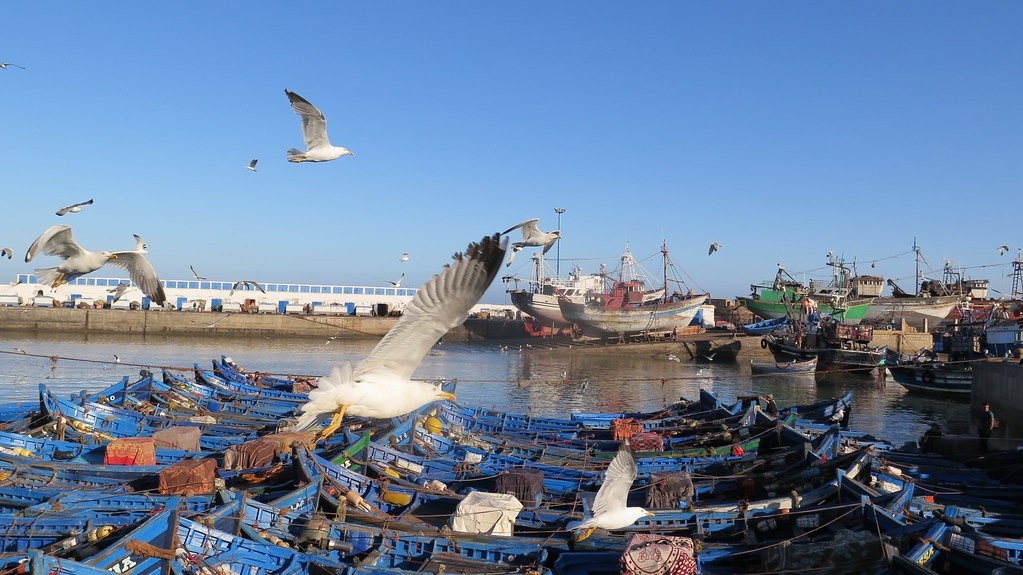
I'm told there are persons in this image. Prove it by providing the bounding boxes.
[671,326,678,342]
[922,423,941,451]
[978,403,994,460]
[760,394,779,416]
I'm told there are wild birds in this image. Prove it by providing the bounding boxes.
[112,355,122,364]
[565,436,655,542]
[281,232,511,443]
[188,265,266,327]
[709,242,723,257]
[383,219,562,288]
[665,353,753,375]
[245,158,259,173]
[24,223,167,309]
[284,87,354,163]
[0,199,94,260]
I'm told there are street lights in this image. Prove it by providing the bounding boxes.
[554,206,567,281]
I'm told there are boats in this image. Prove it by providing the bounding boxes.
[741,314,790,336]
[888,235,990,301]
[552,237,710,333]
[749,353,820,376]
[764,269,892,373]
[1,350,1023,575]
[500,237,669,329]
[881,245,1023,396]
[734,250,880,326]
